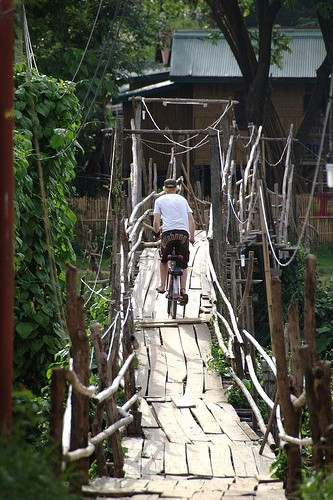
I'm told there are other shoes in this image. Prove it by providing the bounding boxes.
[180,294,189,306]
[157,286,164,293]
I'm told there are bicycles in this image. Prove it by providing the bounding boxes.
[154,232,195,320]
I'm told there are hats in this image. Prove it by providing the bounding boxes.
[164,178,176,188]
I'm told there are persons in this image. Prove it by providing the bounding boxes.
[153,179,195,306]
